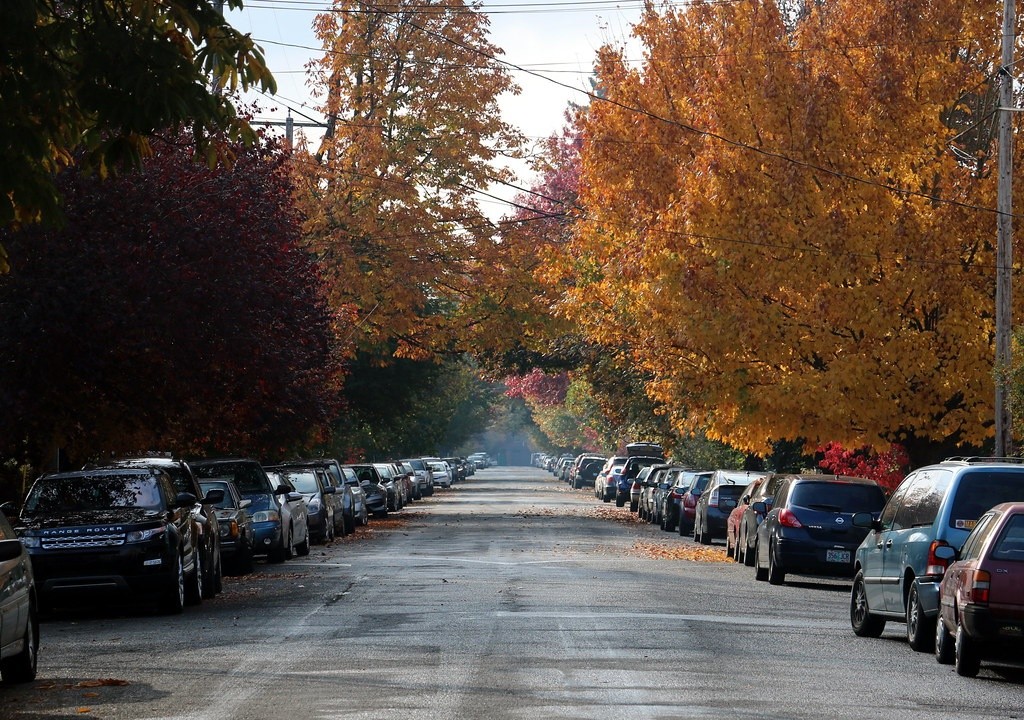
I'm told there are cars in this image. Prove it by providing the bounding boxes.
[754,472,885,585]
[194,475,256,574]
[658,469,699,532]
[933,501,1024,678]
[0,506,41,684]
[627,463,683,525]
[676,470,715,536]
[725,476,766,561]
[737,473,787,566]
[273,450,499,545]
[530,453,628,503]
[260,466,310,557]
[691,468,766,543]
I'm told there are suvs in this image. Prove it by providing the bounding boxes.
[80,457,223,601]
[11,464,204,616]
[615,442,667,507]
[185,457,292,565]
[850,455,1024,652]
